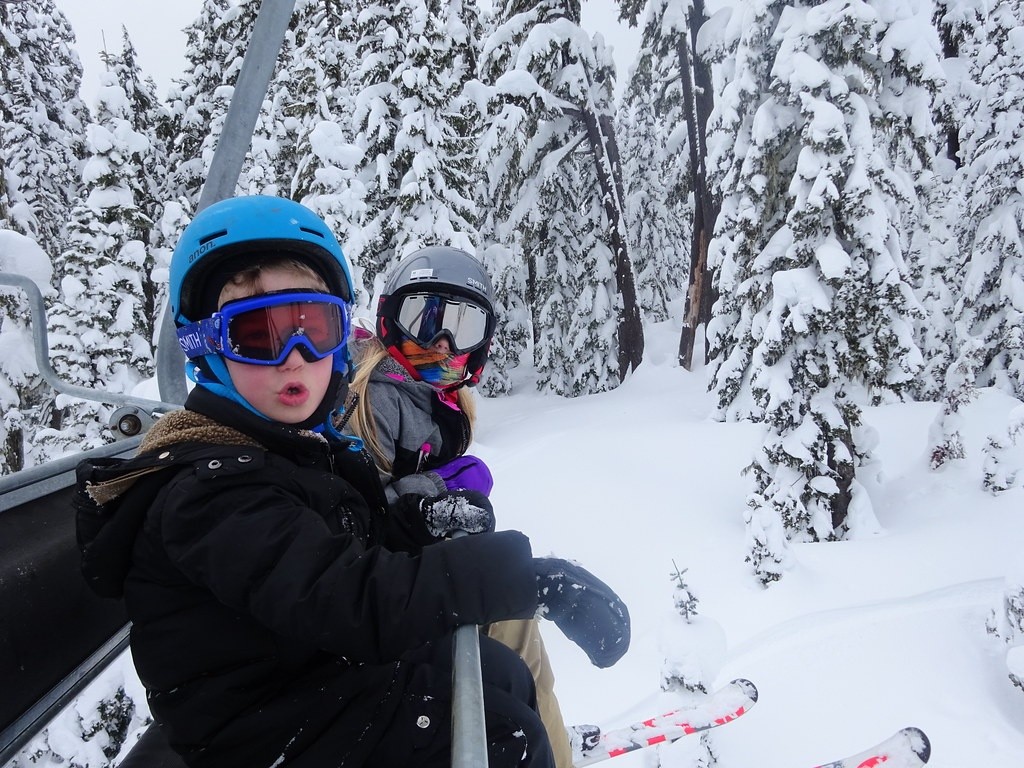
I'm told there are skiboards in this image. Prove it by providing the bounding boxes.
[567,677,932,768]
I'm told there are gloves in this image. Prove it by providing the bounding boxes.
[533,558,632,669]
[419,491,496,538]
[434,455,493,497]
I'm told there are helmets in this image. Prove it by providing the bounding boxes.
[169,194,356,381]
[376,246,497,374]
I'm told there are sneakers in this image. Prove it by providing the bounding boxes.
[564,724,600,752]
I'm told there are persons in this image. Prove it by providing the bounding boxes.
[74,196,631,768]
[326,243,597,768]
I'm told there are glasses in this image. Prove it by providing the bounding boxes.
[394,291,493,353]
[215,289,352,366]
